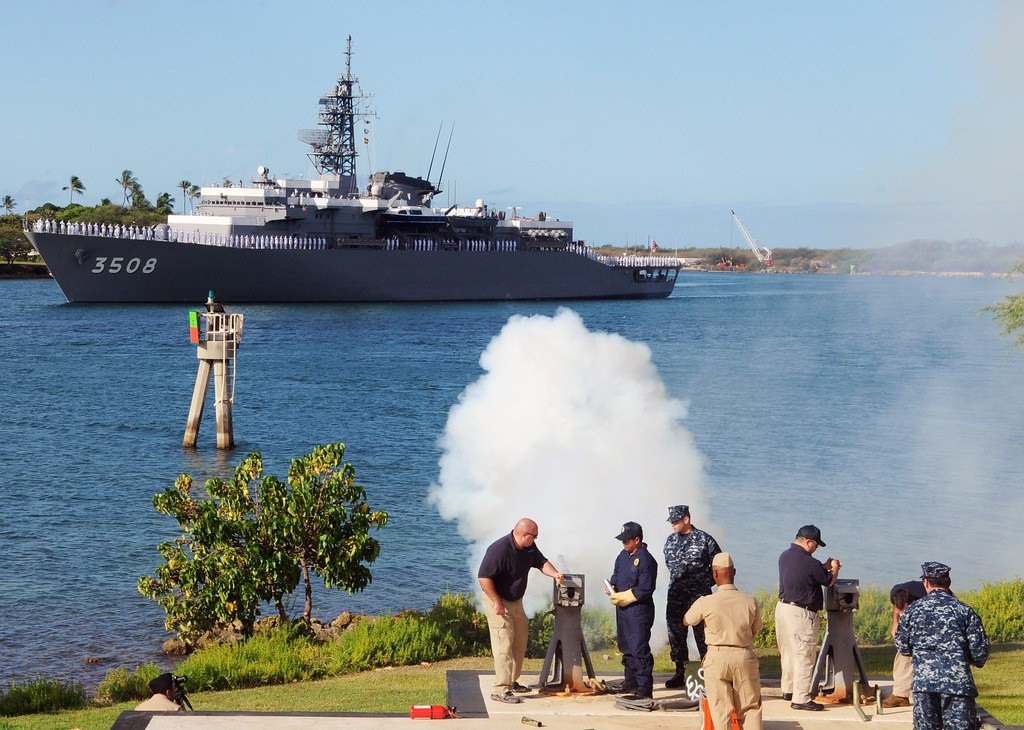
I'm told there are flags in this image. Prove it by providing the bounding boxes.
[651,241,658,253]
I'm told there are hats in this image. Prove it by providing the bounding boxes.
[919,561,951,579]
[713,551,733,568]
[798,524,826,547]
[614,521,643,541]
[667,504,690,522]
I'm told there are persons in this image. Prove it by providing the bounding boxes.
[881,581,958,708]
[662,504,723,688]
[683,552,762,730]
[774,524,842,711]
[892,562,990,730]
[478,517,565,704]
[133,672,185,712]
[605,521,658,701]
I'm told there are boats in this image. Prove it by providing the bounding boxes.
[381,205,447,229]
[21,34,686,303]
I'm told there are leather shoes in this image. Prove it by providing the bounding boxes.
[609,680,639,694]
[621,692,645,701]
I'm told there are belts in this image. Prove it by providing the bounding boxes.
[780,598,818,613]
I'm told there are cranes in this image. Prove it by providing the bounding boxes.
[730,210,773,267]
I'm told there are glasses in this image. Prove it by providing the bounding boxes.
[807,538,819,549]
[525,532,538,539]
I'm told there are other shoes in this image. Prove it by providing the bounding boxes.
[790,700,824,711]
[783,692,792,700]
[881,694,910,708]
[665,670,685,688]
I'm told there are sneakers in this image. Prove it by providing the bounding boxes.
[490,691,520,703]
[508,681,532,693]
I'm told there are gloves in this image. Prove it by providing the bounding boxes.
[609,588,638,606]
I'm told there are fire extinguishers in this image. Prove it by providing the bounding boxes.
[410,705,458,719]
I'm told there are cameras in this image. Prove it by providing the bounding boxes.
[171,674,188,687]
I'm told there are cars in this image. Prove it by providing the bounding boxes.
[690,259,701,265]
